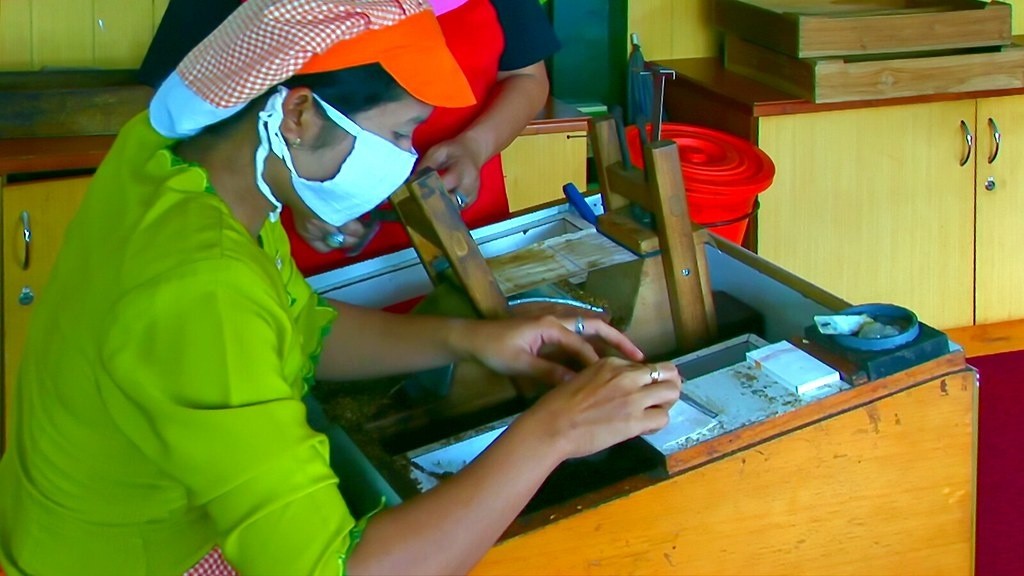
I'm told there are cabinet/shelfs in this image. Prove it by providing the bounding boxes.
[647,59,1024,359]
[0,69,587,466]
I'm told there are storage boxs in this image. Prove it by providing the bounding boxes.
[713,0,1024,104]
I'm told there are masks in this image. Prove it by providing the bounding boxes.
[280,94,417,226]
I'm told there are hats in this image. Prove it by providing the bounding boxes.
[150,0,478,137]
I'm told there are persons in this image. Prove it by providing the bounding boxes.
[0,94,682,576]
[137,1,561,311]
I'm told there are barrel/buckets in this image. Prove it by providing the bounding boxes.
[625,122,776,247]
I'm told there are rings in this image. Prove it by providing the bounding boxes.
[574,313,584,336]
[644,362,660,384]
[452,190,468,210]
[324,232,345,248]
